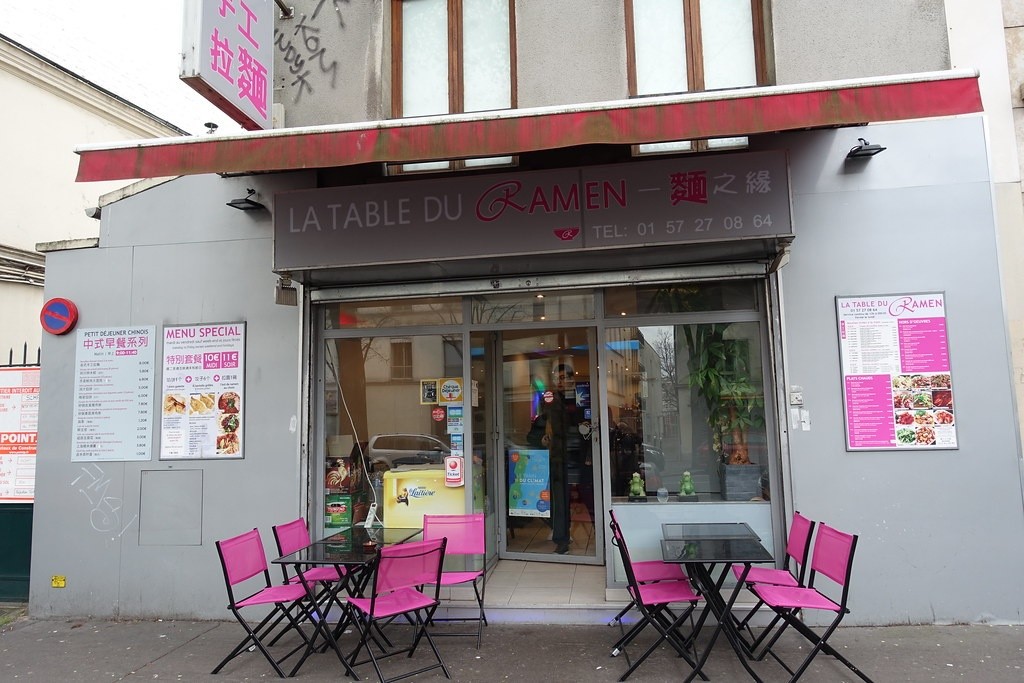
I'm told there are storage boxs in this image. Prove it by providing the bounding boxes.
[325,493,368,526]
[325,457,362,493]
[324,526,370,554]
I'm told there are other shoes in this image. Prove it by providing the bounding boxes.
[570,537,574,544]
[553,544,570,555]
[547,529,554,541]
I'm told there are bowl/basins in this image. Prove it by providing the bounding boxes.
[363,542,377,550]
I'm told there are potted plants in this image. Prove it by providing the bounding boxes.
[683,323,766,501]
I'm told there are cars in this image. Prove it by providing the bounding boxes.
[757,469,771,501]
[549,428,665,482]
[364,433,484,474]
[697,431,767,472]
[472,431,532,457]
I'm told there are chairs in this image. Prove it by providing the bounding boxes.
[210,512,489,683]
[607,510,874,683]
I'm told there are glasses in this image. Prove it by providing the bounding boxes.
[551,375,570,382]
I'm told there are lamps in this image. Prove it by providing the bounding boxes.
[226,188,266,211]
[846,138,887,158]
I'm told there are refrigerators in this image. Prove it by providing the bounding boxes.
[382,461,488,545]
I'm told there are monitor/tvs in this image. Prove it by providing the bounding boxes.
[574,379,591,408]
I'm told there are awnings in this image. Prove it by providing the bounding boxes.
[73,71,986,182]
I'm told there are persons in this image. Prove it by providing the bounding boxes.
[527,364,595,554]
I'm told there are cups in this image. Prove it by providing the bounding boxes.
[657,487,668,502]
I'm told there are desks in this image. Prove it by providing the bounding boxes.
[661,522,762,651]
[272,543,399,681]
[660,539,777,683]
[321,527,423,648]
